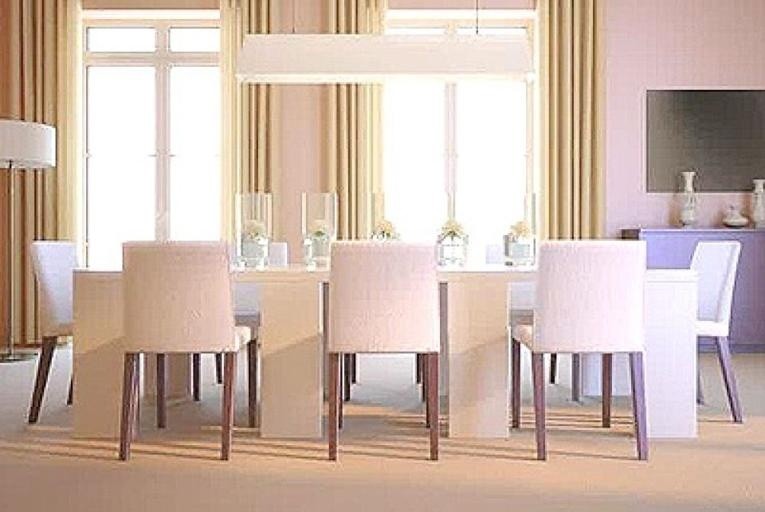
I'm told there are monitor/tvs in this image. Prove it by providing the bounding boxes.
[646,90,765,193]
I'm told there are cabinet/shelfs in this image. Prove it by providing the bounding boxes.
[620,224,765,354]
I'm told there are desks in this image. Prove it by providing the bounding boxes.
[66,266,703,445]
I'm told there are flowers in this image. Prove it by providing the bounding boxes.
[240,215,531,241]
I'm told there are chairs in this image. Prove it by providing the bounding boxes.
[23,237,85,424]
[681,236,747,426]
[505,235,655,466]
[322,233,441,468]
[114,238,256,464]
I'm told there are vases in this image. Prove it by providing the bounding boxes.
[750,179,765,228]
[240,234,533,268]
[676,171,699,229]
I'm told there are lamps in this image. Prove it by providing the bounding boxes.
[228,0,542,86]
[1,111,58,366]
